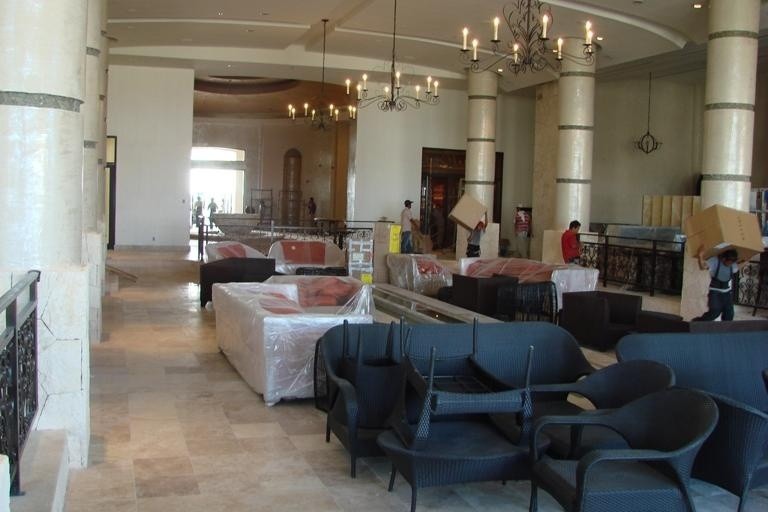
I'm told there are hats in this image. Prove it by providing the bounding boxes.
[405,200,413,204]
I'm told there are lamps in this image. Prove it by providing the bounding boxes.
[633,70,664,155]
[283,17,357,130]
[344,1,440,113]
[456,1,595,75]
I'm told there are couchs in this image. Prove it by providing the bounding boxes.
[198,241,686,409]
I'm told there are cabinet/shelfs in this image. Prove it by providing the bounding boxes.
[278,189,305,219]
[250,187,274,223]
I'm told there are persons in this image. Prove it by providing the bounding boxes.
[691,244,749,322]
[194,196,205,227]
[401,200,418,253]
[466,212,488,257]
[308,198,316,216]
[561,221,583,264]
[207,198,219,229]
[513,206,530,260]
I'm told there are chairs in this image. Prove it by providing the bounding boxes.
[313,316,768,512]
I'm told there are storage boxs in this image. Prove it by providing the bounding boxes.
[448,192,488,233]
[684,204,764,263]
